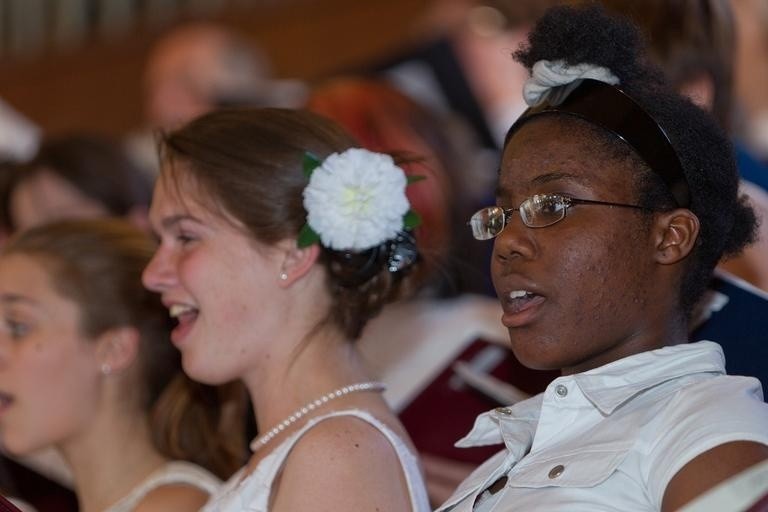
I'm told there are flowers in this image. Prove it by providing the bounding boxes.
[292,132,427,253]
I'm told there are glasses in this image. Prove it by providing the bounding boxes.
[465,193,704,248]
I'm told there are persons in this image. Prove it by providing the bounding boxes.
[1,0,768,511]
